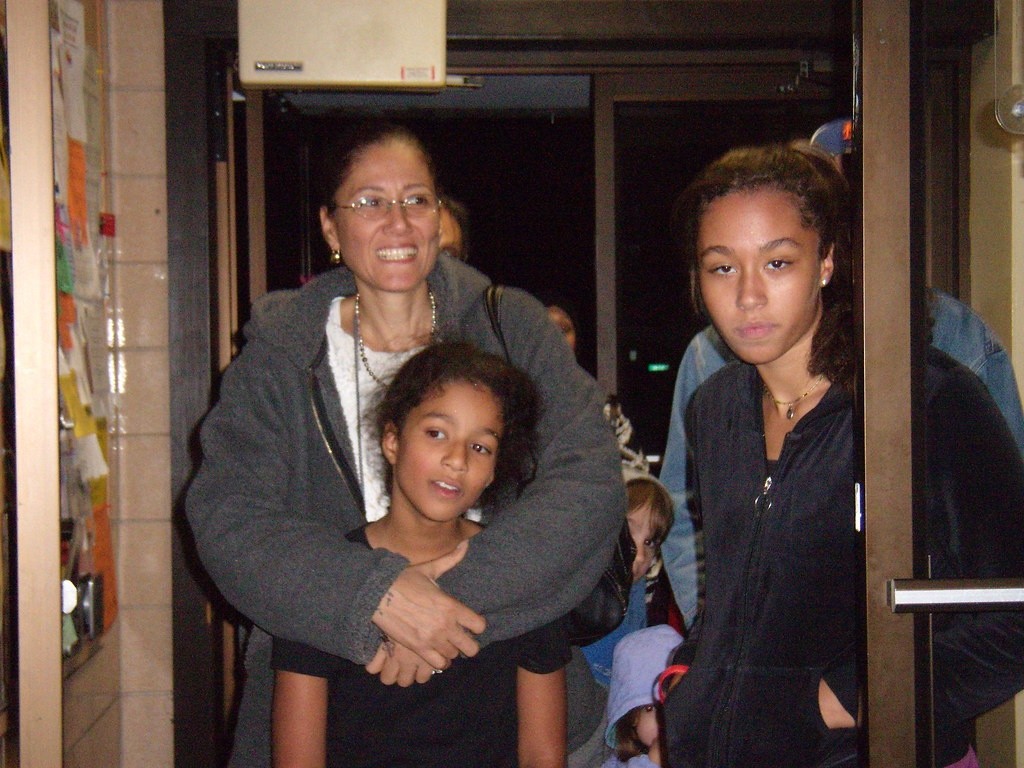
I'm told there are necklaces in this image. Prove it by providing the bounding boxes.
[353,288,436,496]
[763,375,825,419]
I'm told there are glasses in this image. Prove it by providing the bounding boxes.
[336,195,443,220]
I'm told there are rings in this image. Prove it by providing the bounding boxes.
[432,669,442,674]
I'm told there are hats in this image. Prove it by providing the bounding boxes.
[811,119,855,155]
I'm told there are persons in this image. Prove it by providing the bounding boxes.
[658,146,1024,768]
[434,201,682,767]
[657,119,1024,626]
[270,342,572,768]
[186,120,629,768]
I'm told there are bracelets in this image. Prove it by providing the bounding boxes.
[658,665,689,703]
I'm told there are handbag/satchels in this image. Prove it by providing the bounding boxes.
[482,277,638,646]
